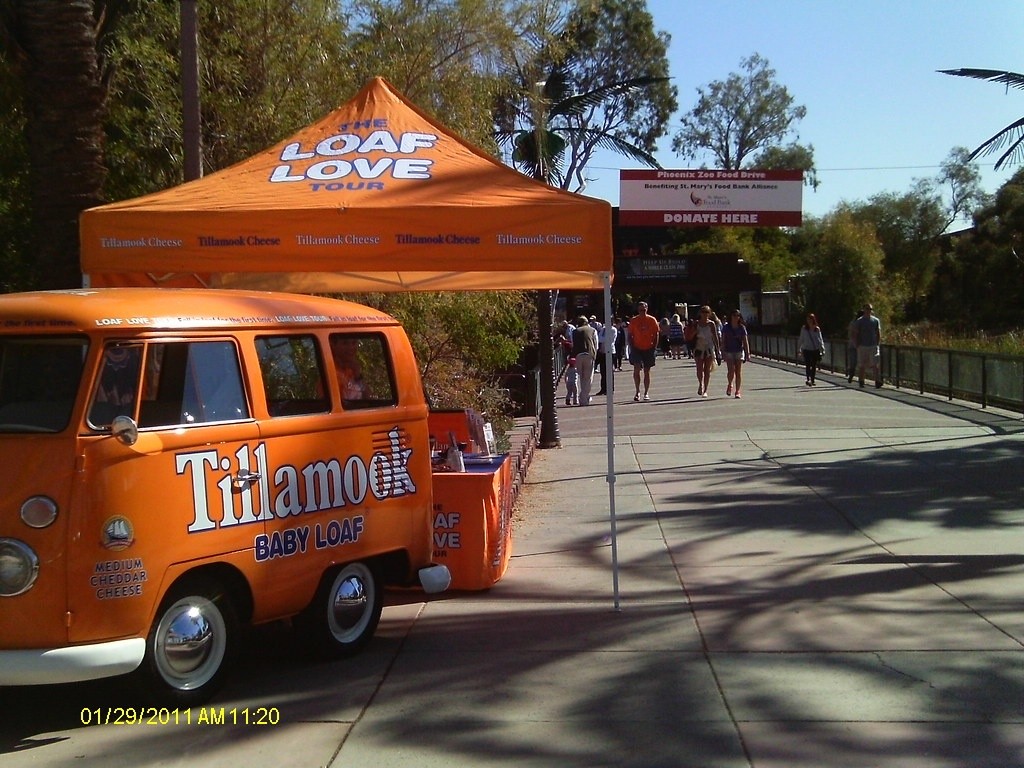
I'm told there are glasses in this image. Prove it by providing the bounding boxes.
[701,311,710,313]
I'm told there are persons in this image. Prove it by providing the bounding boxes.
[596,316,617,395]
[614,315,635,371]
[659,314,698,359]
[797,314,825,385]
[317,338,369,400]
[564,358,580,405]
[710,312,747,366]
[568,316,599,405]
[559,320,575,354]
[847,304,883,388]
[627,302,660,401]
[688,306,719,398]
[722,309,750,398]
[590,315,601,331]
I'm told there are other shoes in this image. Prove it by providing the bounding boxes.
[588,397,593,403]
[703,392,708,398]
[643,393,649,400]
[596,390,607,396]
[615,368,618,371]
[806,381,816,387]
[726,384,733,396]
[618,366,622,371]
[572,402,579,405]
[859,381,864,387]
[634,392,641,401]
[698,384,703,395]
[848,374,854,383]
[595,369,598,372]
[734,390,740,399]
[664,351,694,360]
[875,381,883,389]
[565,402,571,405]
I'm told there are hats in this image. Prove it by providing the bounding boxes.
[577,315,588,322]
[568,358,576,364]
[589,315,596,319]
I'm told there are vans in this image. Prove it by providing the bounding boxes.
[0,288,432,705]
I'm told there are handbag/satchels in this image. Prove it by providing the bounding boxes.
[685,320,699,341]
[817,348,822,361]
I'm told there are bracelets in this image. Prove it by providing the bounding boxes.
[747,353,750,354]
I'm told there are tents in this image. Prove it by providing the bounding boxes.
[79,77,619,614]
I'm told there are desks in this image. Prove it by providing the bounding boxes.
[432,452,512,590]
